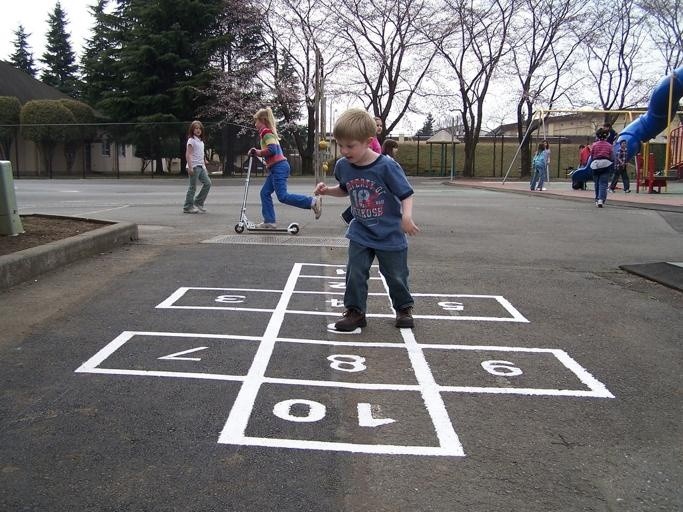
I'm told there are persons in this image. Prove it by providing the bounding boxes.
[338,138,399,227]
[530,142,547,191]
[317,107,421,332]
[608,139,631,194]
[577,144,592,168]
[248,107,323,229]
[541,140,550,181]
[589,127,614,208]
[604,123,617,143]
[182,120,211,213]
[367,116,383,154]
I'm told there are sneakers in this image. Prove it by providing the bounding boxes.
[596,200,604,208]
[340,213,349,226]
[608,188,631,193]
[311,196,323,220]
[396,307,413,328]
[183,206,199,213]
[256,222,277,228]
[194,196,207,211]
[337,312,367,331]
